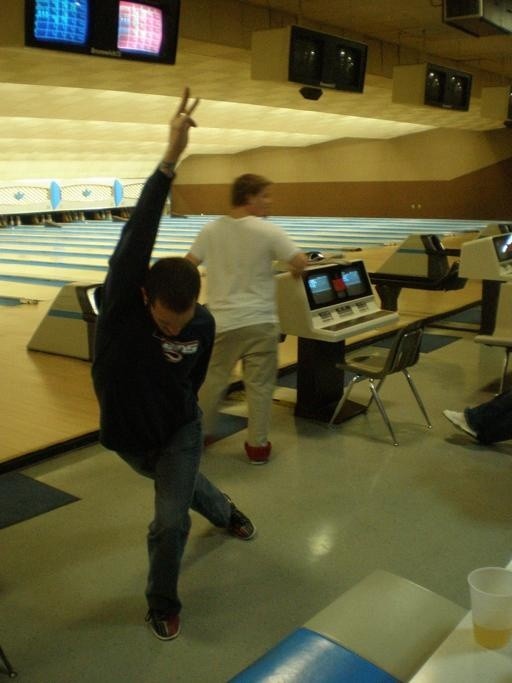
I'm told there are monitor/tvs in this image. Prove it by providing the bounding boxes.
[319,30,369,94]
[302,270,340,311]
[90,0,183,67]
[391,62,449,111]
[491,233,512,263]
[445,68,473,112]
[0,0,95,61]
[249,25,327,89]
[337,261,371,298]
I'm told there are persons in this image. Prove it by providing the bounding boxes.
[91,85,255,636]
[441,391,510,446]
[185,172,307,465]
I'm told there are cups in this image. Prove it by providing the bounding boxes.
[468,567,512,650]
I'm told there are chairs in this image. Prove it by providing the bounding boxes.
[328,321,433,444]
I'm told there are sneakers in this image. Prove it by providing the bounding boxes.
[145,612,181,640]
[224,493,256,540]
[245,443,271,464]
[442,410,477,440]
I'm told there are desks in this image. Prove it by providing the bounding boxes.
[289,283,481,425]
